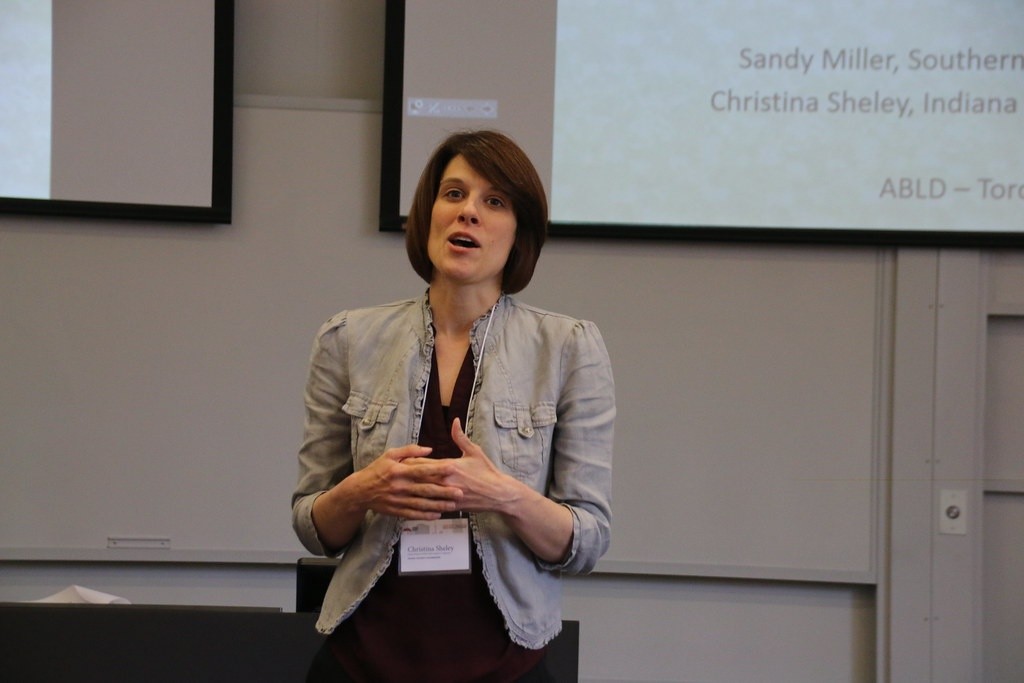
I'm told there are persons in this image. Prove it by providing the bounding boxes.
[290,129,616,683]
